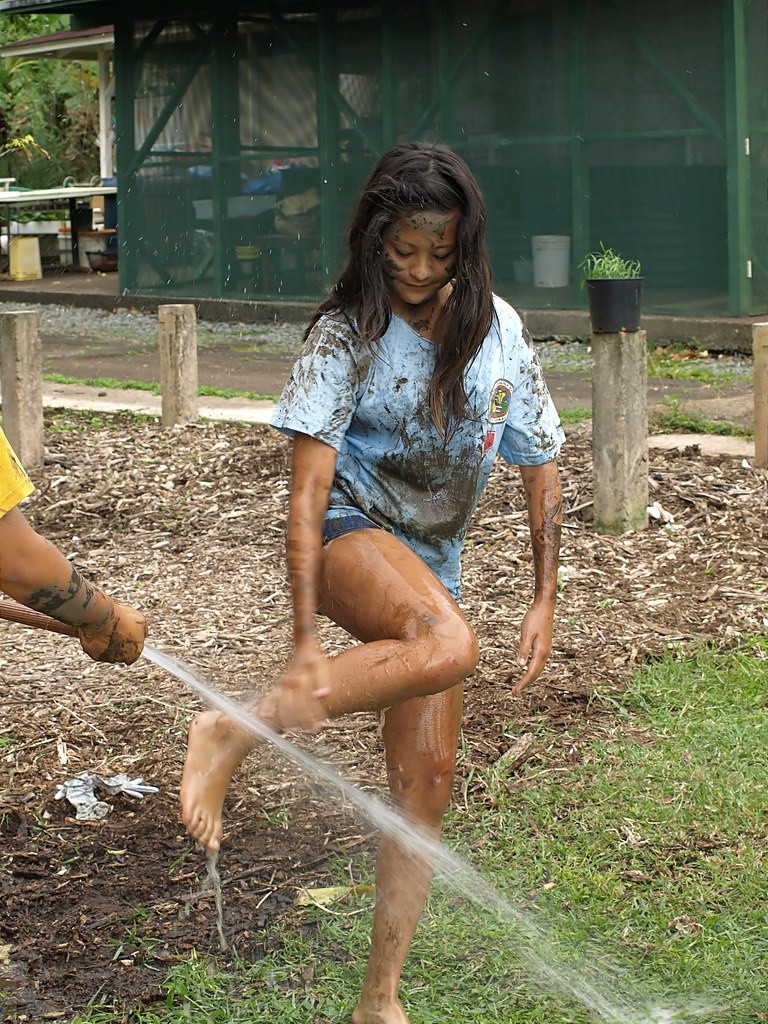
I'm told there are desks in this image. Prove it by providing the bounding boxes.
[0,186,118,265]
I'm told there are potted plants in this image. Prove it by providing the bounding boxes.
[575,241,643,334]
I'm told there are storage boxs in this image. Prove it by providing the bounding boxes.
[9,237,42,282]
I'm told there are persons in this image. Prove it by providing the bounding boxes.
[1,426,149,666]
[179,143,567,1024]
